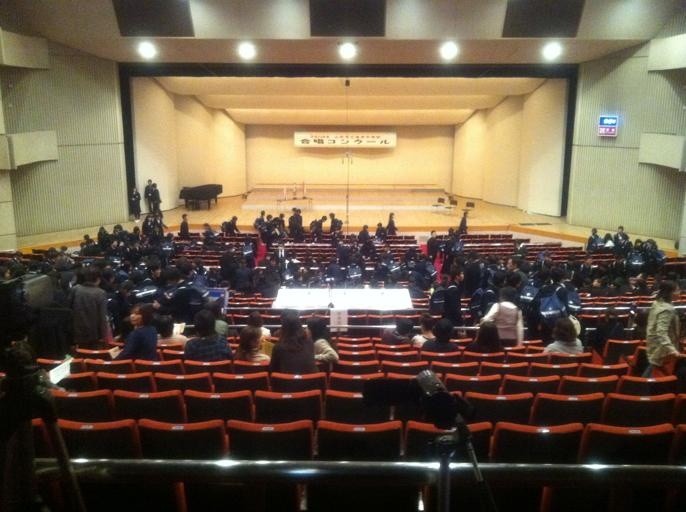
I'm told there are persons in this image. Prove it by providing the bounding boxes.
[144,179,154,213]
[1,208,399,375]
[151,184,163,218]
[130,187,142,223]
[1,341,60,414]
[393,211,686,367]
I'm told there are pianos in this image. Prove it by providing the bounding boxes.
[179,184,221,209]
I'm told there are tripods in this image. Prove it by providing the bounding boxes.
[0,388,88,512]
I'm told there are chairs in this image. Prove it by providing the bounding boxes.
[429,195,476,218]
[0,225,686,512]
